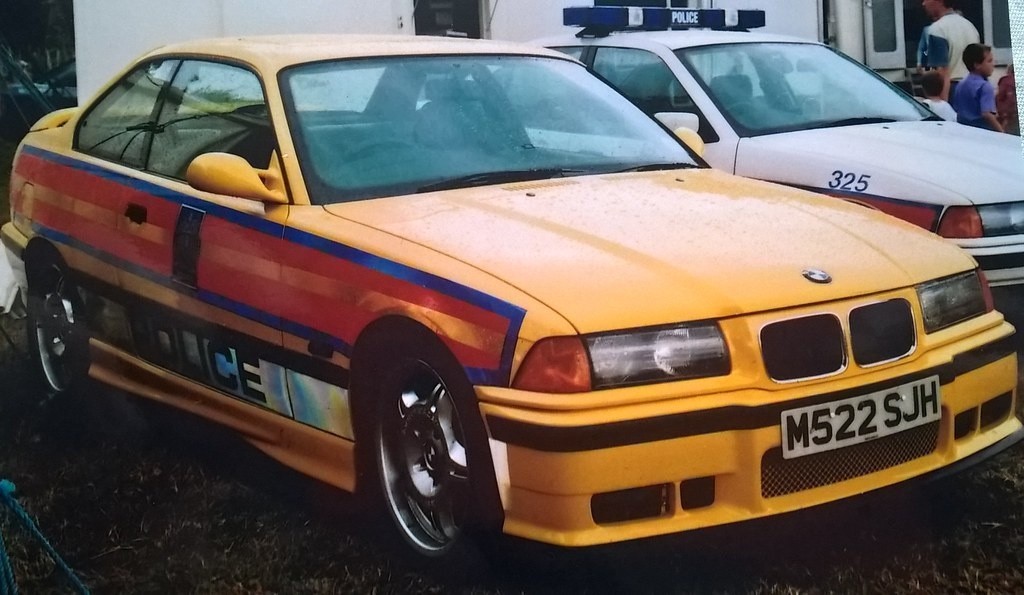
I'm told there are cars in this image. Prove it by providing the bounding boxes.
[0,34,1023,585]
[0,58,77,111]
[416,6,1024,287]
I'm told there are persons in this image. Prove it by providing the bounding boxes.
[995,63,1020,136]
[917,0,980,107]
[952,43,1004,133]
[920,72,958,122]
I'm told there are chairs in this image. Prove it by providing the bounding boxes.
[710,72,759,118]
[416,82,492,155]
